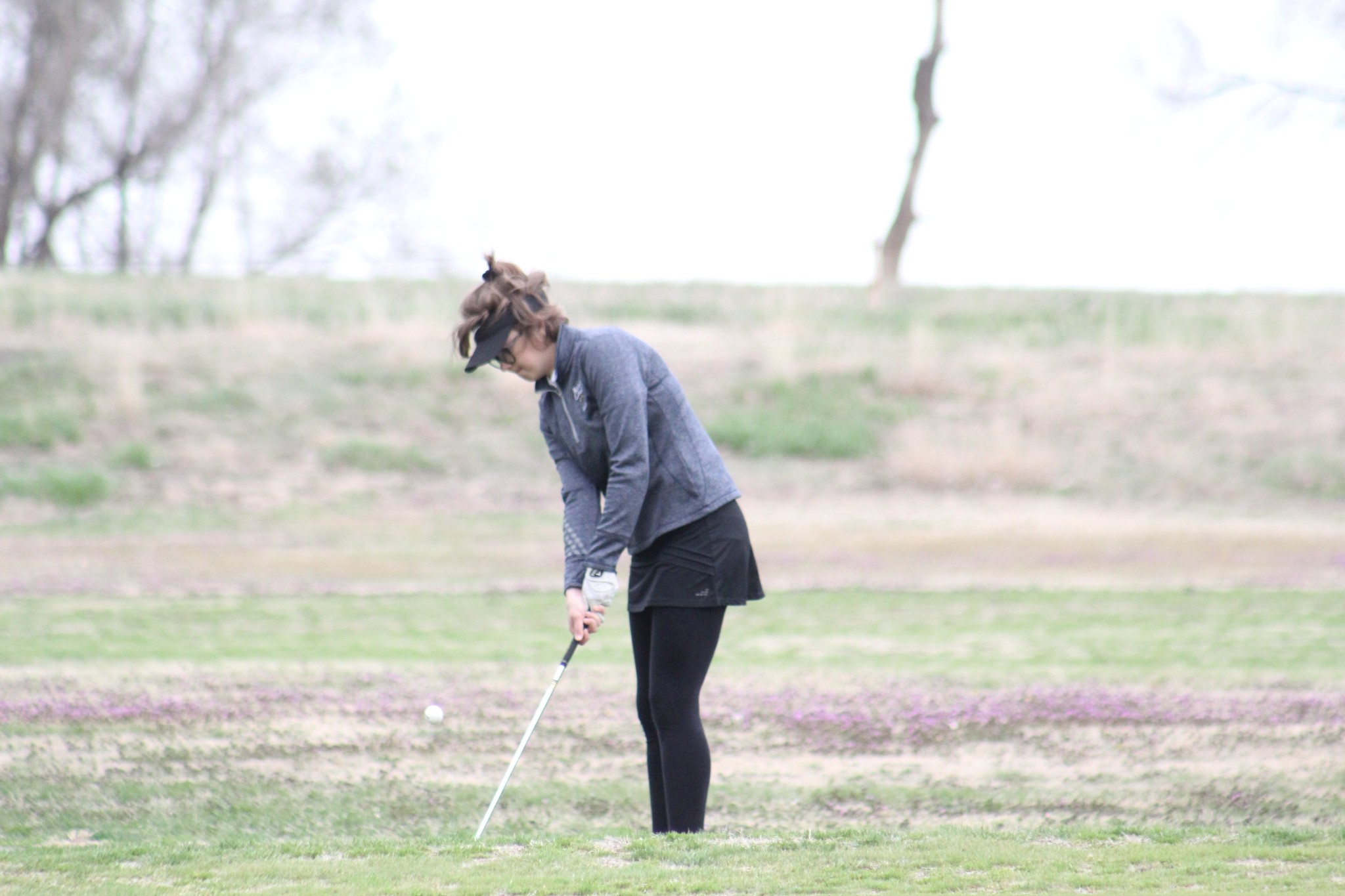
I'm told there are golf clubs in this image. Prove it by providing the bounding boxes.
[471,605,591,844]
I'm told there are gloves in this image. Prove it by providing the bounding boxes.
[582,567,617,623]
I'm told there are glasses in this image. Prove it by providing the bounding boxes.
[488,333,522,369]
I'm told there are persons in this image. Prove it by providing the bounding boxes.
[456,255,765,834]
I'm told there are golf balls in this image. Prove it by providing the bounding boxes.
[423,704,444,725]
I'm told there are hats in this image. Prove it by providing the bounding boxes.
[464,290,549,373]
